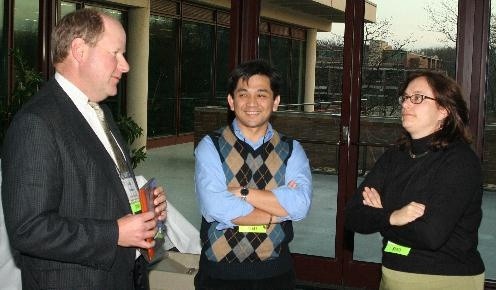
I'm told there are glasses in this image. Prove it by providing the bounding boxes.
[398,94,437,104]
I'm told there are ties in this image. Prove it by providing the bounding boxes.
[88,102,132,178]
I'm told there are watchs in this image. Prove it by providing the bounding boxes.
[240,186,249,201]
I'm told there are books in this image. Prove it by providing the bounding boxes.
[140,178,166,260]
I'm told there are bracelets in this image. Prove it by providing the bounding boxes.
[268,215,272,228]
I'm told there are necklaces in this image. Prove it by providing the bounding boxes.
[408,148,431,159]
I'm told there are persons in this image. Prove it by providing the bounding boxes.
[347,72,485,290]
[194,59,314,290]
[0,8,166,290]
